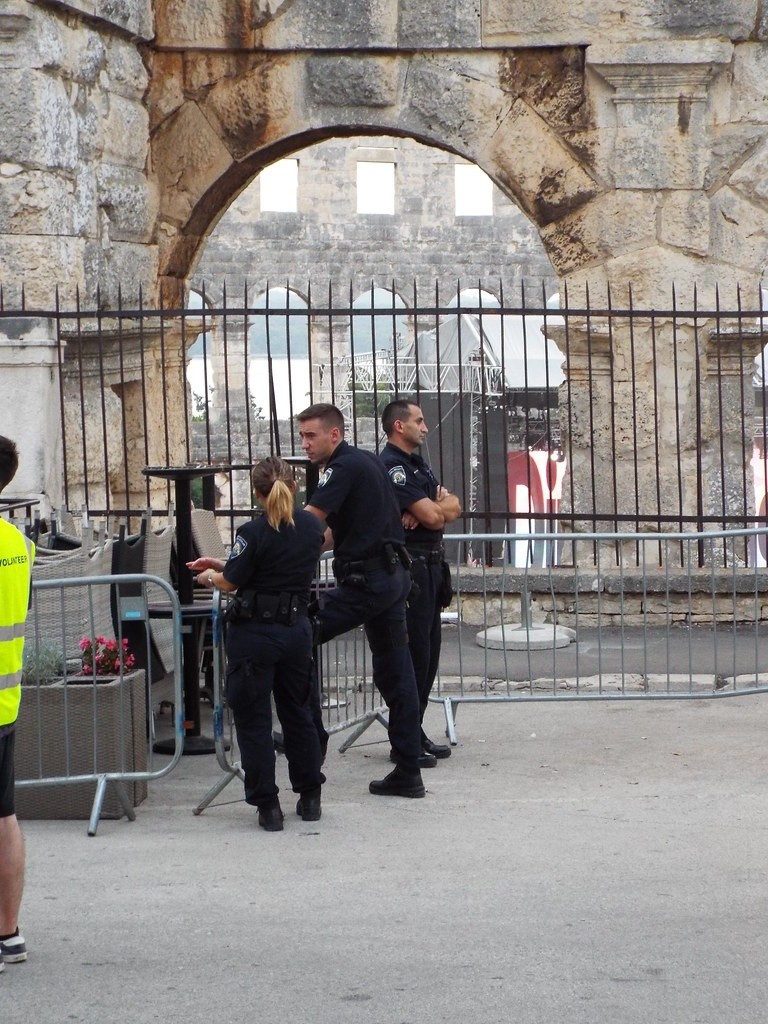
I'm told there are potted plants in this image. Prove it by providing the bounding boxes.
[13,642,133,820]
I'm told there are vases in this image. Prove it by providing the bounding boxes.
[76,669,148,806]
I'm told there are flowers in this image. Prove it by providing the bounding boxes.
[79,636,135,675]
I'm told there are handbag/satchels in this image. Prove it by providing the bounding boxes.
[440,559,453,608]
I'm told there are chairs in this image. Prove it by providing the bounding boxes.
[23,499,226,680]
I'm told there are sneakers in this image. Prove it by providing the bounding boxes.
[0,935,27,973]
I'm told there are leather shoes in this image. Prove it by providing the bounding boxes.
[390,746,436,767]
[255,804,283,831]
[369,761,425,798]
[423,738,452,759]
[296,797,320,821]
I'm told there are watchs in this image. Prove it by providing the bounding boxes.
[207,572,214,587]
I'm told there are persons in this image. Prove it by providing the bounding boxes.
[186,402,425,832]
[379,399,462,769]
[0,435,37,972]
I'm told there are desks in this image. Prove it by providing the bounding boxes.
[149,602,230,756]
